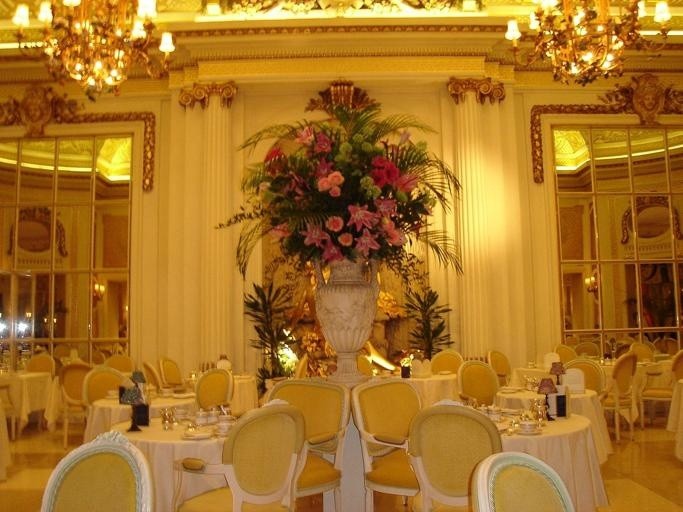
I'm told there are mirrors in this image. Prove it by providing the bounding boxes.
[551,124,683,358]
[0,131,134,358]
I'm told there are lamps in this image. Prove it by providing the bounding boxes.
[11,0,178,103]
[503,0,673,89]
[584,275,598,294]
[92,281,106,299]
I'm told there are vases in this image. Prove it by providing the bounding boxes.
[314,253,376,379]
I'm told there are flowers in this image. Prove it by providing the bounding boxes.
[222,77,460,270]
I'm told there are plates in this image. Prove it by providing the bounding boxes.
[214,432,229,436]
[184,432,211,439]
[516,430,543,435]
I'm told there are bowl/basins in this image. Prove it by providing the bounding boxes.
[519,421,536,432]
[218,421,233,433]
